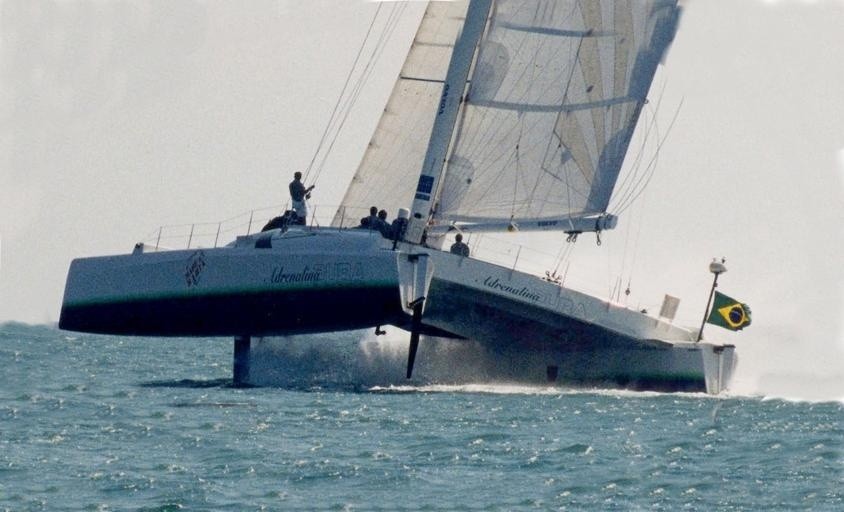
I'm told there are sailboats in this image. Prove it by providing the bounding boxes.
[57,0,739,396]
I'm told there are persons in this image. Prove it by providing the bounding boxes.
[288,171,315,227]
[357,205,429,246]
[450,234,469,256]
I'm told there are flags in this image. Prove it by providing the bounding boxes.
[706,289,752,332]
